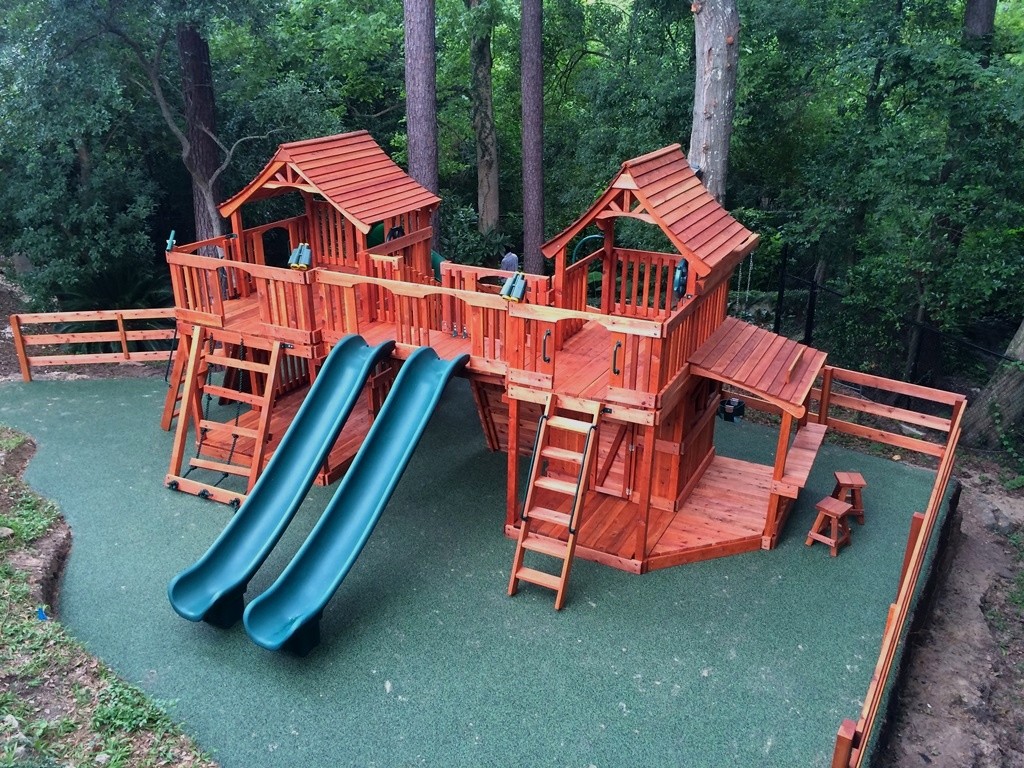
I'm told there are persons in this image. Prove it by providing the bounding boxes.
[501,247,518,286]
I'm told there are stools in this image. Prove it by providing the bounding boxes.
[830,471,867,526]
[805,495,854,556]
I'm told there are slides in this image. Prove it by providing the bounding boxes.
[242,346,472,652]
[169,336,396,623]
